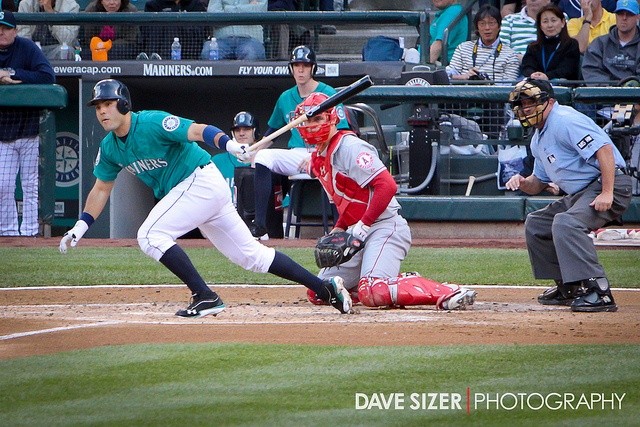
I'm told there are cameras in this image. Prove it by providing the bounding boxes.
[467,70,491,86]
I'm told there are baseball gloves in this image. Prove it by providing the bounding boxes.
[314,231,365,274]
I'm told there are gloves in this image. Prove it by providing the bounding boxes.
[218,135,252,163]
[59,212,95,254]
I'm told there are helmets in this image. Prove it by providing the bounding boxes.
[288,45,317,75]
[290,92,340,153]
[231,111,258,129]
[86,79,132,115]
[509,77,555,139]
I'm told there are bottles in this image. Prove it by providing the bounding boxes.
[170,38,182,60]
[61,42,68,59]
[209,38,219,60]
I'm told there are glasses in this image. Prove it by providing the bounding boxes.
[478,21,497,26]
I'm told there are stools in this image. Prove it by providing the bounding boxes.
[284,173,335,239]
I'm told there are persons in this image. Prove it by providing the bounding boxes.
[210,109,258,226]
[15,0,80,59]
[0,8,57,237]
[320,0,349,33]
[445,4,520,86]
[601,79,640,195]
[567,0,616,54]
[83,0,141,61]
[58,78,353,320]
[199,0,269,60]
[504,76,633,312]
[251,45,350,241]
[581,0,640,87]
[418,0,468,65]
[499,0,569,64]
[137,0,211,61]
[518,4,579,80]
[289,93,478,311]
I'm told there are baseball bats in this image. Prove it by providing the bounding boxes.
[235,74,374,156]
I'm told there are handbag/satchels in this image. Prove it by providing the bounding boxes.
[362,36,403,61]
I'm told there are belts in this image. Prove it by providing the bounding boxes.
[598,166,632,175]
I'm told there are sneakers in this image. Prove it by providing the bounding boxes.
[571,277,618,312]
[401,271,420,278]
[436,281,476,310]
[315,276,352,314]
[174,293,225,319]
[538,280,583,304]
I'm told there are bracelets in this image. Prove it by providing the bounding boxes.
[582,19,591,24]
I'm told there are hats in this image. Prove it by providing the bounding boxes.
[614,0,640,15]
[1,9,14,27]
